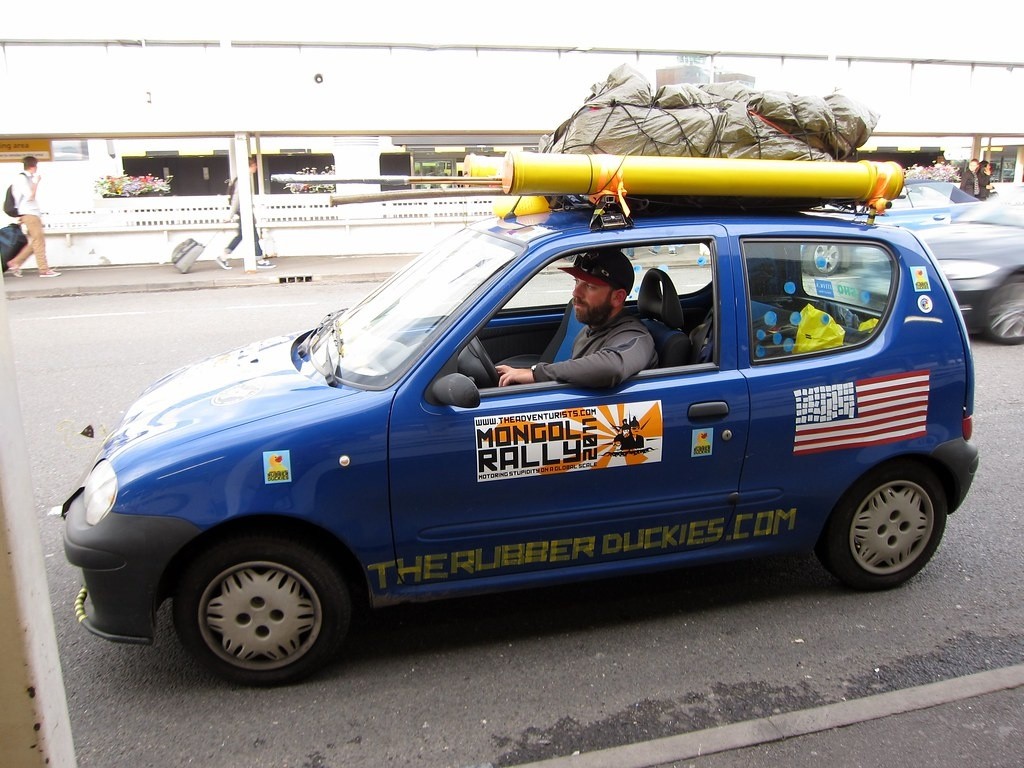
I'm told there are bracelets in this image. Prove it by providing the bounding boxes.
[531,365,536,375]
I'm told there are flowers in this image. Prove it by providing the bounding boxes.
[93,170,172,196]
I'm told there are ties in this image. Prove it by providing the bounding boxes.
[972,171,980,195]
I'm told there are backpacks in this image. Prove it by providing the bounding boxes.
[3,173,28,217]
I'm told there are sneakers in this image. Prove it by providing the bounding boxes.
[257,260,277,268]
[40,269,62,277]
[7,261,23,278]
[216,256,232,269]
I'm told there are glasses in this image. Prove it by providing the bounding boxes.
[573,254,610,278]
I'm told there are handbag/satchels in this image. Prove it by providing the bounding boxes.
[792,302,845,354]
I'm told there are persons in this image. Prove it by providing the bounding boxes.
[961,159,980,198]
[216,157,277,269]
[6,156,61,277]
[978,160,995,201]
[494,250,658,388]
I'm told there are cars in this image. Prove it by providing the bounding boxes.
[832,179,981,231]
[858,190,1024,346]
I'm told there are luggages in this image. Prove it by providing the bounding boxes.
[171,218,231,273]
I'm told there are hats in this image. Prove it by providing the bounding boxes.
[557,249,635,295]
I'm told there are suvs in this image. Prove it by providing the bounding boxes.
[60,153,978,689]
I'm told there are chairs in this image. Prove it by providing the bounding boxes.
[637,268,691,367]
[495,296,588,368]
